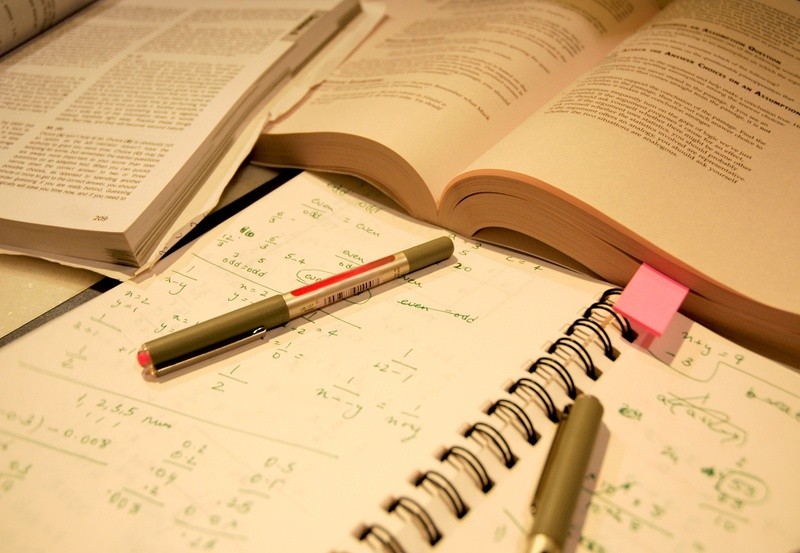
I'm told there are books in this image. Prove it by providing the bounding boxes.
[3,168,800,553]
[247,0,800,365]
[0,0,390,282]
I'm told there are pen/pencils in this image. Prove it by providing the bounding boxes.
[520,395,605,553]
[136,234,455,381]
